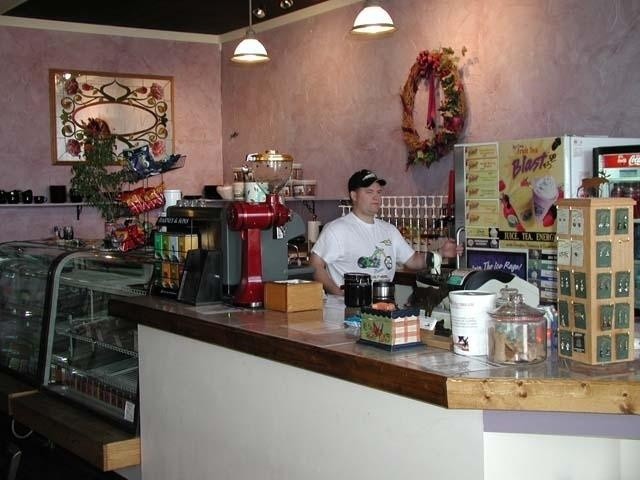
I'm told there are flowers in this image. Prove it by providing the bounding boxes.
[62,77,167,157]
[399,47,466,169]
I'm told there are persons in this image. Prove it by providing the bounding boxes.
[309,169,464,296]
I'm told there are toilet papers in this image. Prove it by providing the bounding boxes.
[307,220,323,244]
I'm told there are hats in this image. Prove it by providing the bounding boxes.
[348,169,387,187]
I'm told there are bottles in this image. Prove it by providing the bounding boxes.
[56,225,74,247]
[381,217,446,254]
[233,168,245,199]
[612,182,639,218]
[487,296,547,366]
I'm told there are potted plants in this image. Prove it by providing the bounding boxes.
[70,134,134,247]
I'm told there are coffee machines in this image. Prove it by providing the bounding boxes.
[228,148,292,310]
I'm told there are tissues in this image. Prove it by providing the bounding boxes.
[264,280,323,314]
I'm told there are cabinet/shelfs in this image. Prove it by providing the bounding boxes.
[0,239,150,434]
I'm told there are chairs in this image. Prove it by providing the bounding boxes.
[0,436,24,480]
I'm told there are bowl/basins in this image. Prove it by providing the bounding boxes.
[218,186,232,200]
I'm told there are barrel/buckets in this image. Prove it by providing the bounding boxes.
[163,188,182,213]
[449,291,498,356]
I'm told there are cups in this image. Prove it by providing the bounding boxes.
[176,199,207,208]
[0,189,48,204]
[343,272,371,307]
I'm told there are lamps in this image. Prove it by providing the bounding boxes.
[230,0,272,64]
[350,0,399,36]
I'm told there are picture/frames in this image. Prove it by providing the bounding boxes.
[48,68,176,166]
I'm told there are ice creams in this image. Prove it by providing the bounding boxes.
[532,176,558,199]
